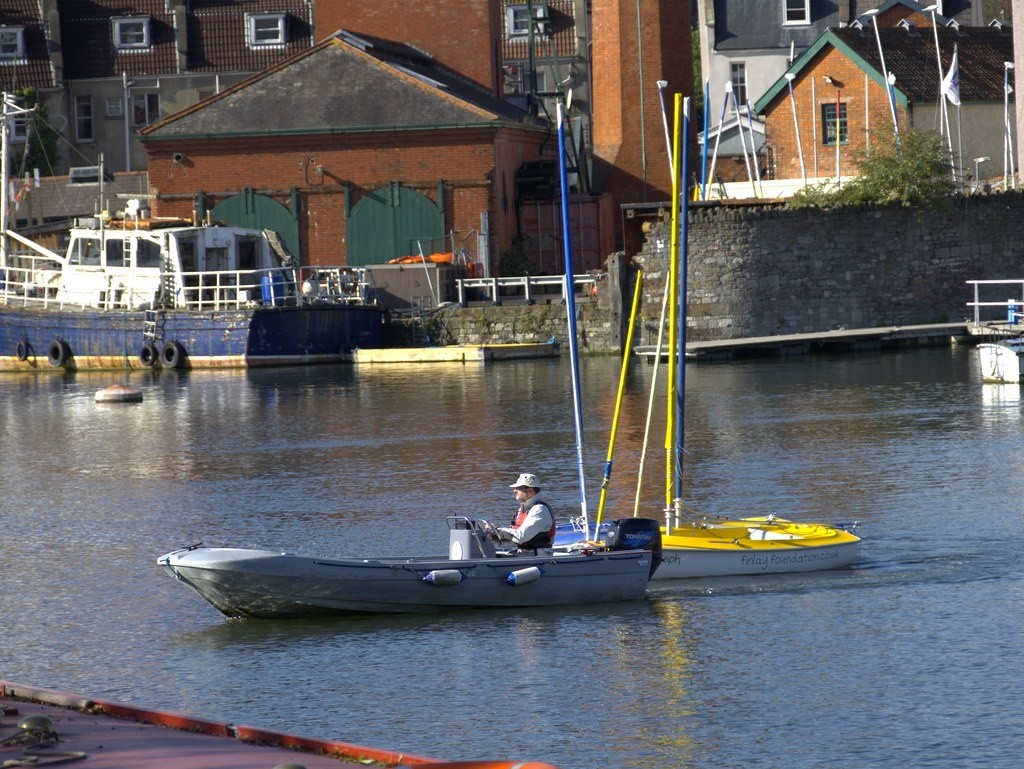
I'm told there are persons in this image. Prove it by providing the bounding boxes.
[485,474,556,558]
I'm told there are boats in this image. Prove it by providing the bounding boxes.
[157,519,664,619]
[552,515,865,581]
[0,200,394,374]
[977,337,1024,385]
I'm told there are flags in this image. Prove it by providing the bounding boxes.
[942,53,961,107]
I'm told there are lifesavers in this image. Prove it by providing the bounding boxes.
[160,342,180,369]
[16,341,28,361]
[48,340,66,368]
[139,345,157,366]
[339,267,358,293]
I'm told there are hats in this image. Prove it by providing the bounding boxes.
[509,471,546,490]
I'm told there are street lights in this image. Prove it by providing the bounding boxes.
[922,4,958,193]
[726,81,757,199]
[657,80,673,182]
[861,8,904,159]
[785,73,809,194]
